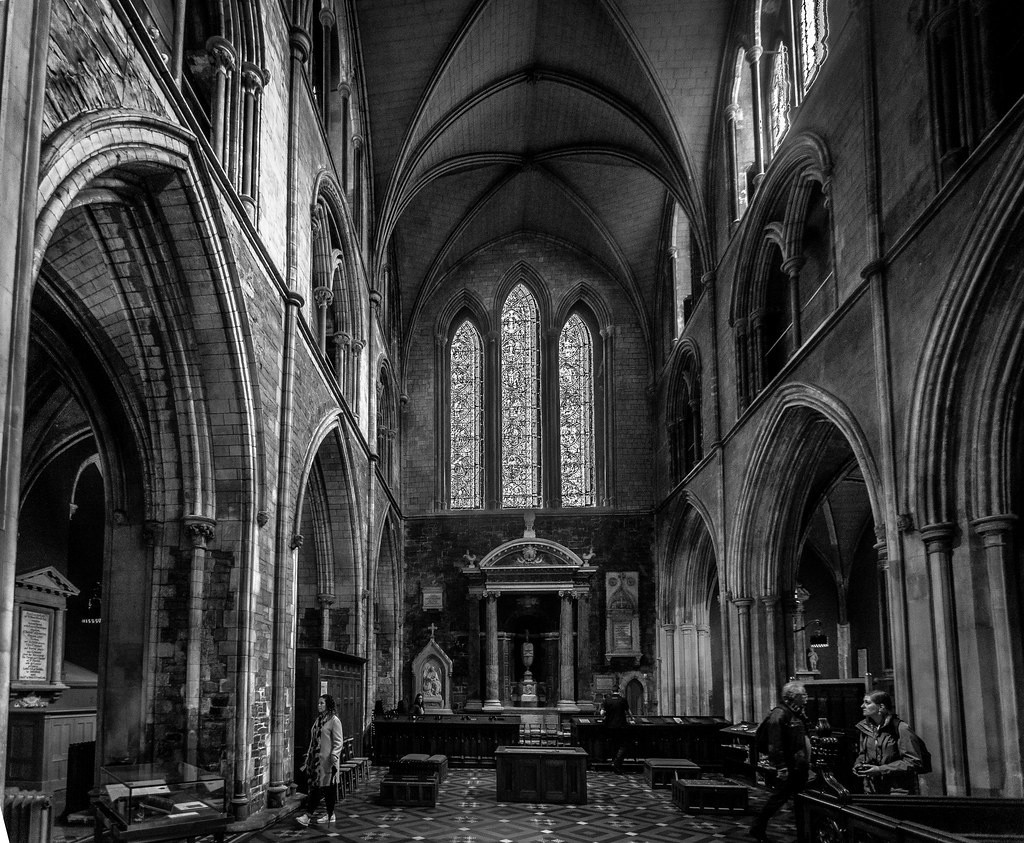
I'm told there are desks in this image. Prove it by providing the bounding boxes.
[495,746,588,805]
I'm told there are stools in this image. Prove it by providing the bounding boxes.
[644,758,702,789]
[399,754,448,783]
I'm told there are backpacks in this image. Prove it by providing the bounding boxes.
[862,717,932,774]
[750,707,790,788]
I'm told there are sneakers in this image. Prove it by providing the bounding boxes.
[295,814,311,826]
[317,813,336,824]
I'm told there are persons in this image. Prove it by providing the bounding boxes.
[808,647,820,672]
[852,690,933,797]
[600,685,635,773]
[749,681,820,843]
[296,694,343,826]
[408,693,425,718]
[422,663,442,697]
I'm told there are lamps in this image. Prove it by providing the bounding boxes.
[793,620,830,648]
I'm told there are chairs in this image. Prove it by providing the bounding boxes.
[336,739,369,801]
[520,723,572,746]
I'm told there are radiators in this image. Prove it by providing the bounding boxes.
[3,787,54,843]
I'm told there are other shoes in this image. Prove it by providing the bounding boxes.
[749,828,776,843]
[613,758,624,775]
